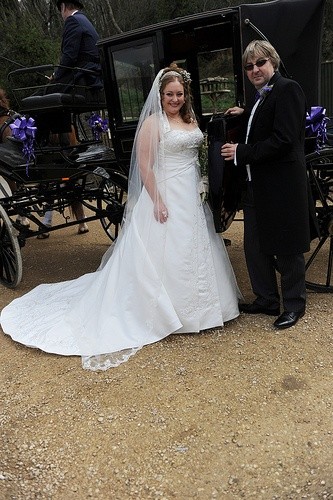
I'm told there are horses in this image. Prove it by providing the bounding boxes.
[0,89,90,241]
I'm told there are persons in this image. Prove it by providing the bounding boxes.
[220,40,312,330]
[132,66,209,326]
[30,0,104,143]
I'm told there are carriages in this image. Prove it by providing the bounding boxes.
[0,63,145,291]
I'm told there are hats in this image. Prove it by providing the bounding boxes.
[56,0,84,11]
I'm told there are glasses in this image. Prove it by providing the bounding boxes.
[243,58,271,70]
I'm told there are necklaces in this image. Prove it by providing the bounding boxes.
[167,115,181,123]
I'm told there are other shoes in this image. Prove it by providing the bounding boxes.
[37,233,50,239]
[78,225,89,234]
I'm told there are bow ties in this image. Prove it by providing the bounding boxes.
[255,92,265,101]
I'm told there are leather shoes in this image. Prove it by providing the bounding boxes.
[273,310,306,329]
[238,302,280,316]
